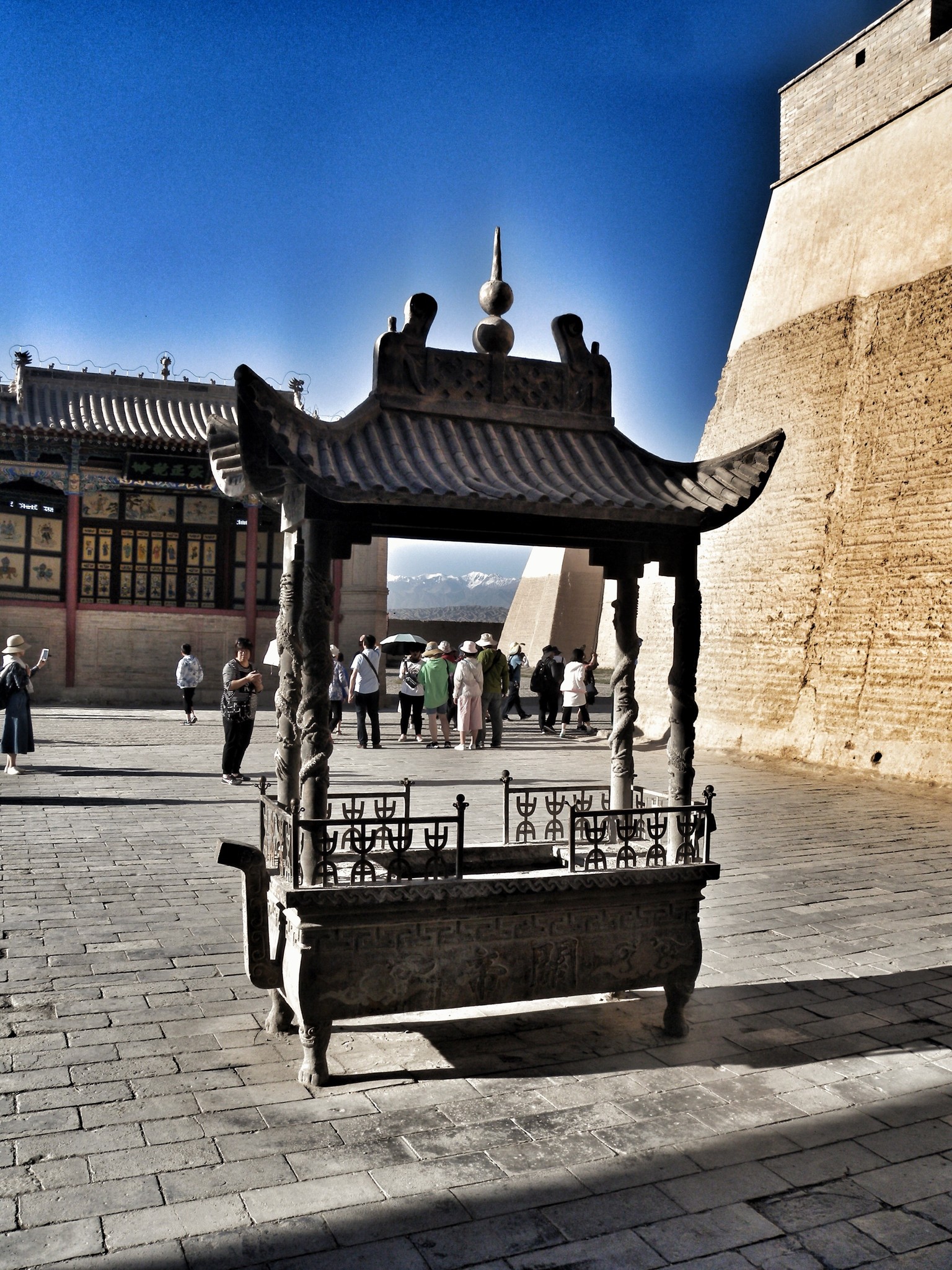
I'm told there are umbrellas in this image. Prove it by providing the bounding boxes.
[379,633,429,675]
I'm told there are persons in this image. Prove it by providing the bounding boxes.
[328,643,352,736]
[0,634,46,775]
[220,637,264,785]
[397,633,534,751]
[529,645,601,739]
[175,643,204,726]
[347,634,382,750]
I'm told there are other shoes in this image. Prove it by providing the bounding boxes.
[415,734,425,742]
[485,716,491,723]
[335,731,342,735]
[357,744,367,749]
[373,744,382,749]
[502,713,532,722]
[542,724,598,738]
[179,716,197,725]
[426,740,502,749]
[4,766,26,774]
[408,723,415,730]
[221,773,251,785]
[398,734,407,742]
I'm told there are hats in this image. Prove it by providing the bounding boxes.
[422,632,499,657]
[577,644,586,652]
[330,644,339,657]
[542,644,557,660]
[1,634,32,654]
[507,642,526,656]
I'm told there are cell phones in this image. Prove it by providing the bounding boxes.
[351,694,354,699]
[42,648,49,661]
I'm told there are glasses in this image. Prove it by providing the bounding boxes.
[235,639,252,647]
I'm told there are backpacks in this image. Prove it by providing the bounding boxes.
[530,659,553,692]
[506,655,518,681]
[554,657,565,680]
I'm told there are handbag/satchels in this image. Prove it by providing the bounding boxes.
[405,675,419,689]
[223,700,252,723]
[586,683,598,697]
